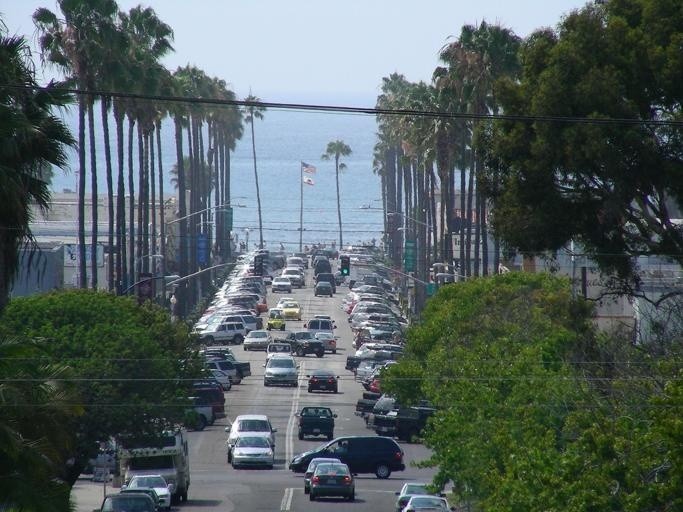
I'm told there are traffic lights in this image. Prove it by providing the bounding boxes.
[342,257,349,276]
[253,257,263,278]
[340,257,350,278]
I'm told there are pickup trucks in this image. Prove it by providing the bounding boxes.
[238,310,263,328]
[295,407,337,441]
[353,322,393,341]
[363,391,380,400]
[345,350,403,377]
[366,406,439,443]
[349,276,392,292]
[188,397,214,430]
[274,331,324,357]
[354,399,375,426]
[257,303,267,317]
[205,354,251,384]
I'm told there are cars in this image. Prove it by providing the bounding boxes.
[282,301,302,320]
[351,327,393,349]
[266,308,285,331]
[394,483,446,512]
[93,492,156,512]
[369,379,380,393]
[314,281,333,297]
[315,333,337,354]
[243,331,273,352]
[309,463,358,502]
[275,298,293,309]
[350,285,384,294]
[121,475,174,512]
[272,276,291,293]
[304,458,341,494]
[313,255,329,268]
[401,496,451,512]
[324,248,338,260]
[306,369,340,393]
[230,436,273,468]
[118,488,165,512]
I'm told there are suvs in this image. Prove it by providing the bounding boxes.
[196,315,257,336]
[224,414,277,463]
[191,322,245,345]
[261,343,297,369]
[289,252,310,269]
[313,273,336,294]
[206,297,256,313]
[311,250,327,266]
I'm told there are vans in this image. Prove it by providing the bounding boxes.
[193,378,220,388]
[372,393,399,415]
[314,315,336,323]
[201,369,230,391]
[344,297,384,314]
[359,315,399,329]
[192,387,225,425]
[355,342,404,357]
[315,260,331,276]
[335,271,345,283]
[303,319,337,333]
[288,436,405,479]
[286,257,304,270]
[286,267,305,286]
[282,269,303,289]
[205,361,236,376]
[198,347,232,354]
[347,301,406,323]
[214,274,266,303]
[261,355,300,387]
[350,313,408,332]
[348,307,406,323]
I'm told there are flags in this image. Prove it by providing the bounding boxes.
[304,178,314,185]
[303,162,316,175]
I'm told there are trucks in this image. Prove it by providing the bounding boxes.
[355,359,397,391]
[116,430,190,504]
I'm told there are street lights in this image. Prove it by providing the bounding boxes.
[121,275,180,296]
[432,263,461,274]
[436,273,466,281]
[122,275,180,299]
[436,272,468,279]
[433,262,457,270]
[133,253,164,267]
[134,254,164,282]
[398,228,413,232]
[386,212,429,227]
